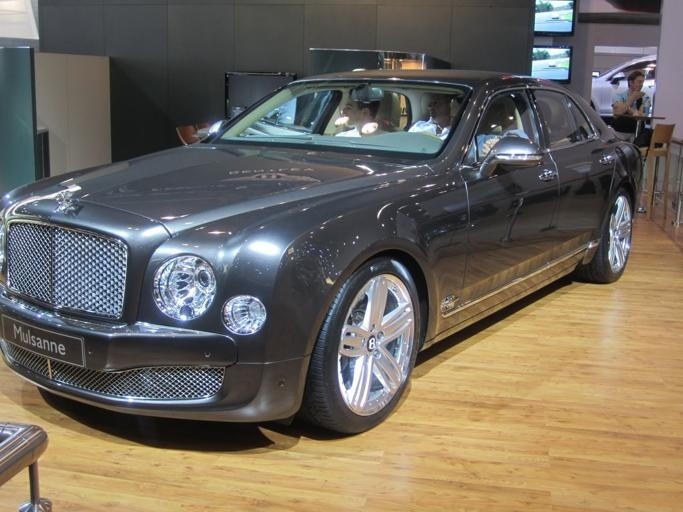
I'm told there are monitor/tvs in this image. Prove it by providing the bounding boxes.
[224,71,296,121]
[533,0,577,37]
[530,46,574,85]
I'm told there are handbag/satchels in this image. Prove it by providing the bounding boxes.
[632,127,662,148]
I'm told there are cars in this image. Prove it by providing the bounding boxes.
[592,55,656,120]
[0,69,642,435]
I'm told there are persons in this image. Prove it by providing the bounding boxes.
[610,72,651,142]
[467,129,529,159]
[407,94,453,141]
[334,86,390,138]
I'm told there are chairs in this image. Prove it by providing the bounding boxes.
[640,123,675,220]
[195,121,210,133]
[176,125,201,145]
